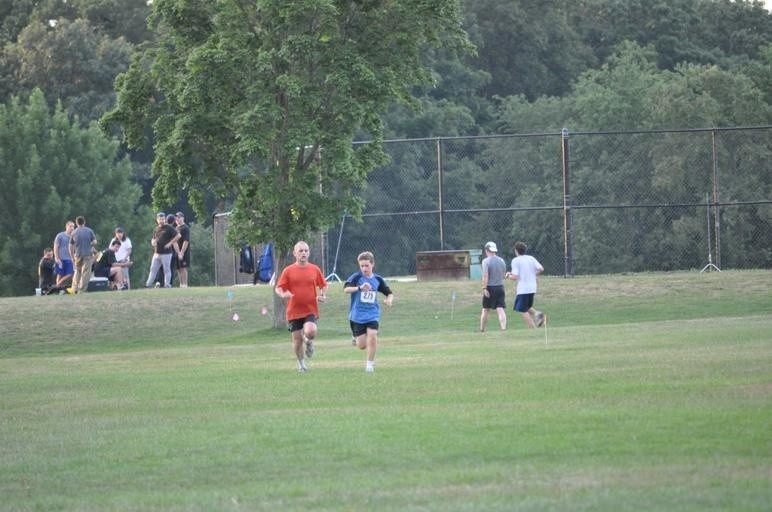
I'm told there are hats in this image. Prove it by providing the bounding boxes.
[484,241,498,253]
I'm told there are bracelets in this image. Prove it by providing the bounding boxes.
[358,286,360,291]
[482,287,487,289]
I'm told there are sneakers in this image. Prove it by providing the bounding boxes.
[303,340,313,359]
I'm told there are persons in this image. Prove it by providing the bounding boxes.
[480,241,507,332]
[275,241,328,372]
[506,242,544,329]
[39,211,189,295]
[344,252,393,373]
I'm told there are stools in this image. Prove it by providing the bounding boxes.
[89,274,108,290]
